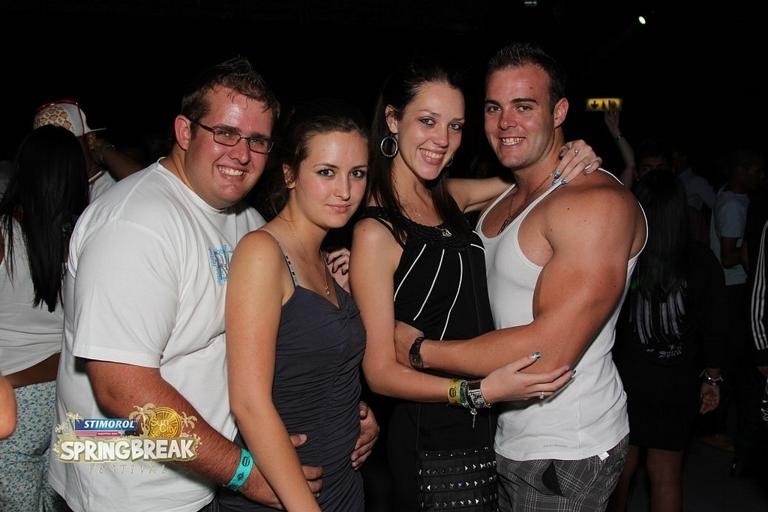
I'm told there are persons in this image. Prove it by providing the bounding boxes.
[219,112,371,510]
[586,97,768,512]
[47,56,382,512]
[0,124,89,512]
[394,45,648,512]
[0,375,18,436]
[348,58,603,512]
[35,103,141,202]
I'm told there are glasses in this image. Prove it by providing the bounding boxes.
[186,115,276,155]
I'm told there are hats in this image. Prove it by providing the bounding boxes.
[33,103,107,138]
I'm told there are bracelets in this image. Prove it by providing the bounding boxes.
[221,447,255,491]
[448,379,493,429]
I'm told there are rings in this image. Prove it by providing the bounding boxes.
[538,393,543,398]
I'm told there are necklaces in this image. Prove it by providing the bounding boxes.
[493,172,555,236]
[410,203,455,237]
[298,239,330,298]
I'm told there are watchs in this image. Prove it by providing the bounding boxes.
[409,336,429,370]
